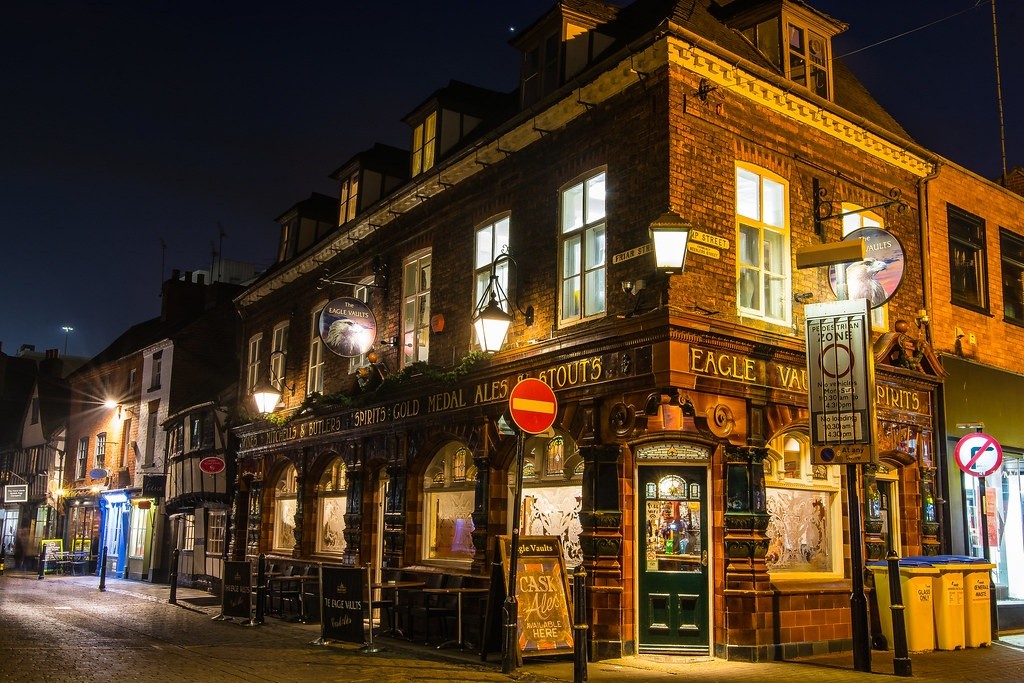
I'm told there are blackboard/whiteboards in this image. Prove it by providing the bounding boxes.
[480,535,574,657]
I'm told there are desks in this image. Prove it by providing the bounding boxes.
[421,588,490,655]
[275,574,320,625]
[363,581,426,641]
[251,571,283,617]
[56,554,82,577]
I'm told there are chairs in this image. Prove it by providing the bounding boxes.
[250,563,322,627]
[51,549,89,577]
[405,574,464,647]
[363,597,393,638]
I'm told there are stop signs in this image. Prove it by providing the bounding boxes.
[509,377,558,435]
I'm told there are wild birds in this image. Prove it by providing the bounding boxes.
[326,319,373,356]
[846,257,887,307]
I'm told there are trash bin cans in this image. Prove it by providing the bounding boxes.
[969,563,997,650]
[866,561,941,652]
[933,563,966,652]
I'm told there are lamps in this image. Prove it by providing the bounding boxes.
[247,346,297,417]
[104,396,121,419]
[469,251,535,357]
[646,200,695,280]
